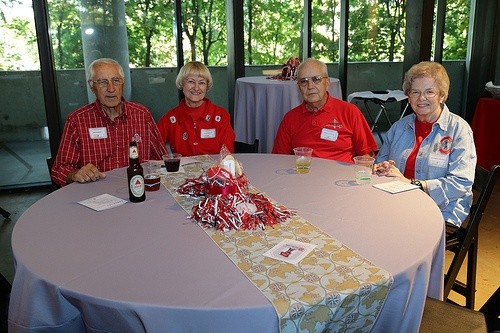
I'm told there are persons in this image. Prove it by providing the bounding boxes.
[371,62,477,239]
[272,58,378,165]
[50,58,169,187]
[155,61,236,157]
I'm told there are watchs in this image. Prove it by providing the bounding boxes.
[413,179,424,191]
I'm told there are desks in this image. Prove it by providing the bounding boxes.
[8,153,445,333]
[470,97,500,171]
[348,90,414,146]
[233,75,342,154]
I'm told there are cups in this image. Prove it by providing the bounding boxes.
[142,162,161,191]
[162,154,182,172]
[353,156,376,185]
[293,147,313,174]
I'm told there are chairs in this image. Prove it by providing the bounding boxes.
[443,163,500,310]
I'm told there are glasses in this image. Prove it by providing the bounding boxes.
[406,88,441,98]
[92,78,123,87]
[297,75,328,87]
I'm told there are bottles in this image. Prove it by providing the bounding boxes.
[127,142,146,203]
